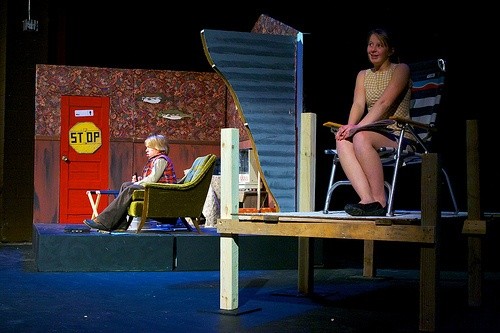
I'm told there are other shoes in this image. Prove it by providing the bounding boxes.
[98,227,127,233]
[83,219,110,230]
[363,200,388,217]
[344,203,367,216]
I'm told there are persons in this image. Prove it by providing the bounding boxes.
[334,29,416,216]
[84,134,177,231]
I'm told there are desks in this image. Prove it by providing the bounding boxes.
[239,191,267,209]
[202,175,221,228]
[85,190,120,220]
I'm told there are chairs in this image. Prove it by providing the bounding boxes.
[126,153,217,234]
[323,76,460,217]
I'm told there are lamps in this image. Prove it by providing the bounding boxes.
[22,0,38,33]
